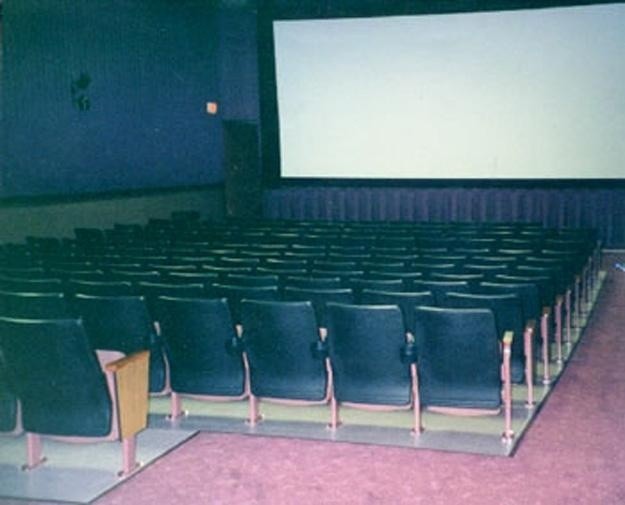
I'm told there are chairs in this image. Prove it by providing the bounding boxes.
[1,213,603,473]
